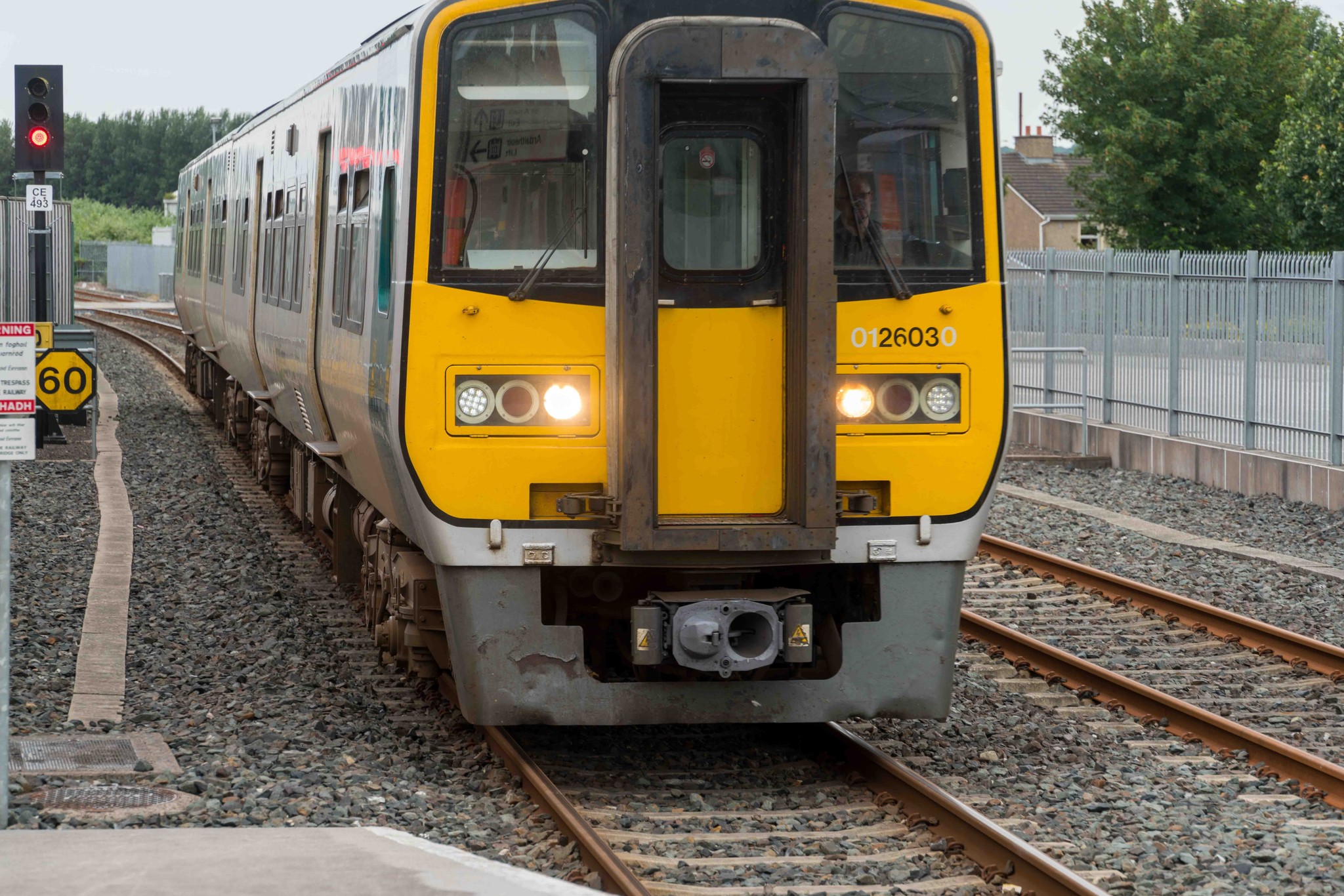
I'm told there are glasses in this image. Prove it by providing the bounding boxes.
[839,190,871,200]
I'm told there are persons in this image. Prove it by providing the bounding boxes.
[835,171,931,266]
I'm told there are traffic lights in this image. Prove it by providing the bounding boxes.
[14,63,65,172]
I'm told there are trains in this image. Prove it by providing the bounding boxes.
[170,0,1008,729]
